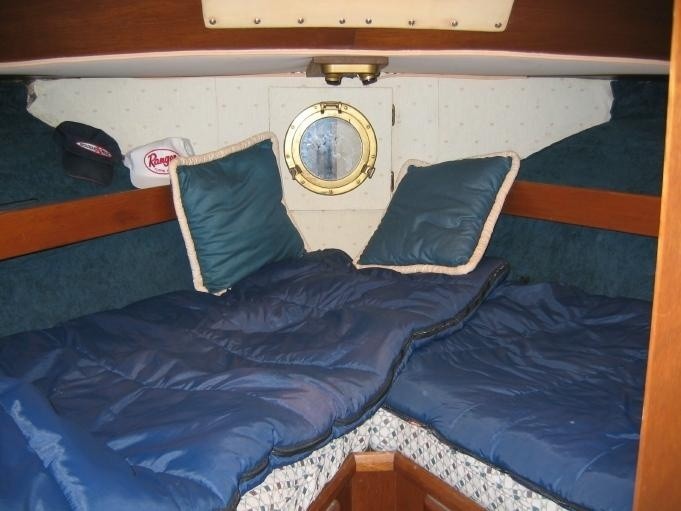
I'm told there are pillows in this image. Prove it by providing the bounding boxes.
[352,149,520,273]
[170,130,311,295]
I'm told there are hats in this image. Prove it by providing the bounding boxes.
[49,121,123,186]
[124,137,195,189]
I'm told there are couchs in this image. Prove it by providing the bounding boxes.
[1,185,662,511]
